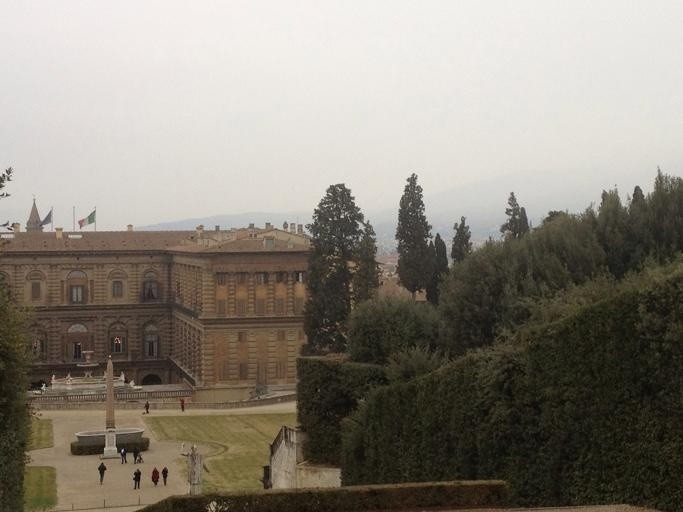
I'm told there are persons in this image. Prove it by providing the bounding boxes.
[152,467,158,486]
[133,446,141,464]
[133,468,141,490]
[161,467,168,486]
[144,400,149,413]
[119,446,128,465]
[180,397,184,412]
[98,462,107,485]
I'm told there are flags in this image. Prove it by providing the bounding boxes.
[40,210,52,226]
[78,210,95,230]
